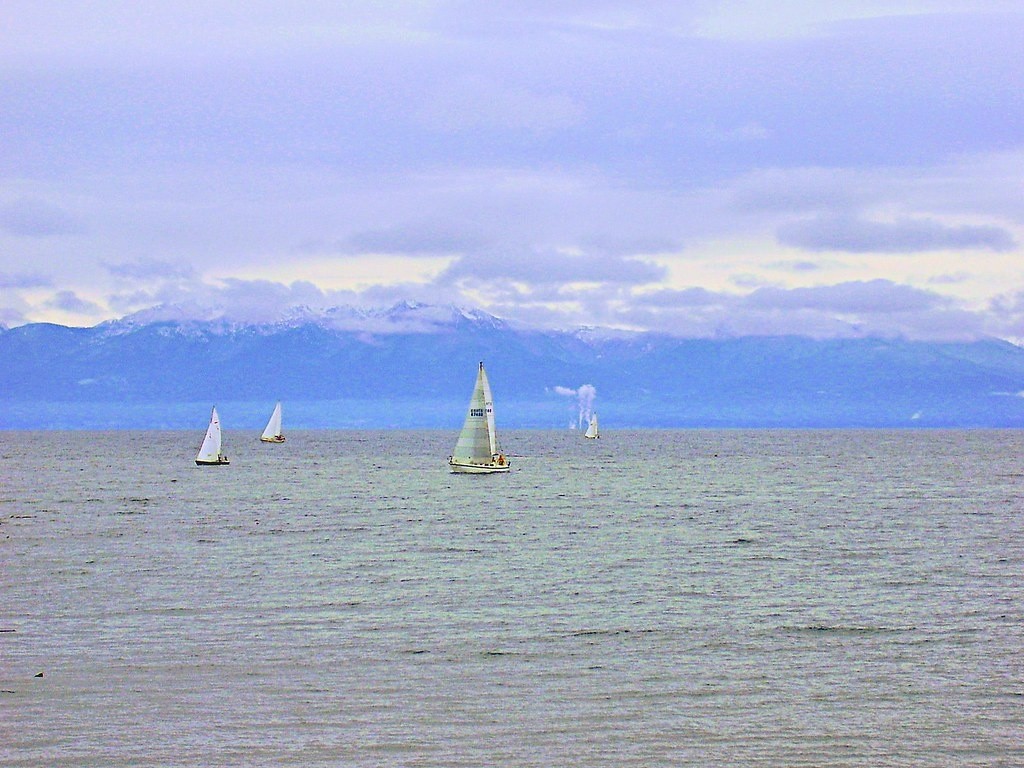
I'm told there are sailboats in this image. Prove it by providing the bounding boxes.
[195,406,231,466]
[451,362,513,474]
[585,413,599,439]
[259,401,287,443]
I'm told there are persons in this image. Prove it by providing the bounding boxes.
[215,454,229,463]
[491,454,507,466]
[274,434,286,441]
